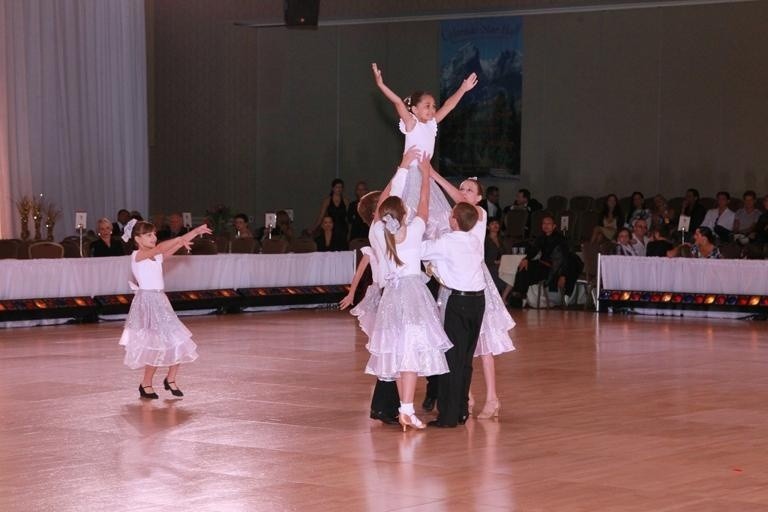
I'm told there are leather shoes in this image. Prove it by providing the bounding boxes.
[370,410,398,427]
[422,390,437,410]
[427,412,469,430]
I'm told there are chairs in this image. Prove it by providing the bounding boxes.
[498,195,768,312]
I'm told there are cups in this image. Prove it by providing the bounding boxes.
[511,247,525,254]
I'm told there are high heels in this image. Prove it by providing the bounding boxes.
[477,396,502,420]
[139,383,160,399]
[398,401,428,433]
[162,377,183,397]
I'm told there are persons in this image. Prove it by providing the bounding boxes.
[118,219,212,399]
[477,188,767,316]
[305,178,370,253]
[339,146,517,432]
[372,62,479,239]
[92,205,295,259]
[139,398,183,431]
[359,416,513,512]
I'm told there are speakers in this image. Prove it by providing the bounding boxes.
[283,0,320,30]
